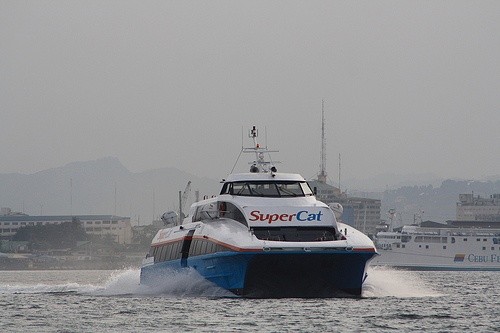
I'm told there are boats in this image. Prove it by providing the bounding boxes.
[369,210,500,269]
[139,124,381,299]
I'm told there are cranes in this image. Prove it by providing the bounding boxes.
[177,180,191,213]
